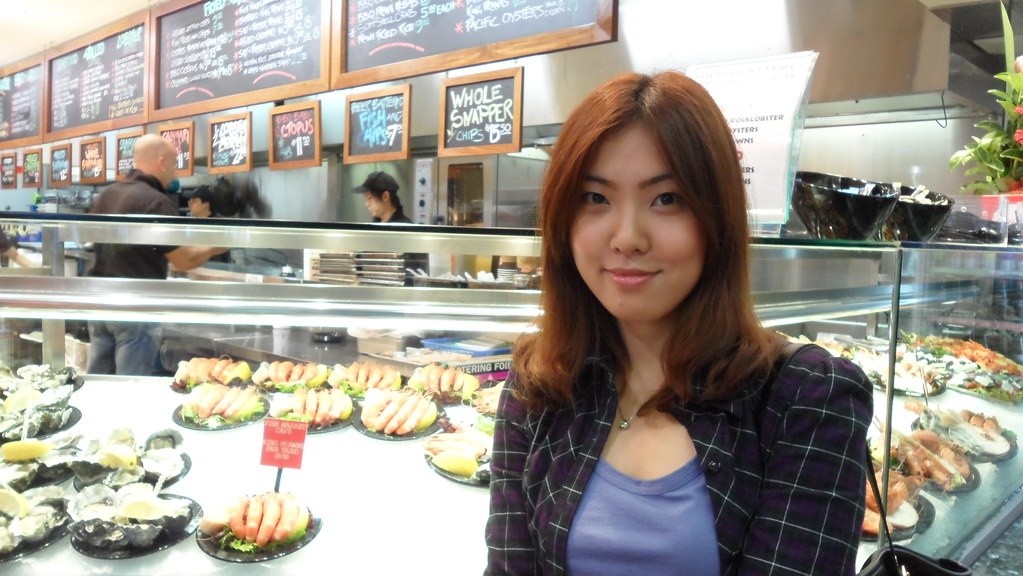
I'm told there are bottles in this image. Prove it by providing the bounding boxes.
[280,264,296,277]
[513,273,543,289]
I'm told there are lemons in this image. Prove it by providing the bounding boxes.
[3,389,43,413]
[0,489,31,518]
[0,440,51,460]
[96,445,136,469]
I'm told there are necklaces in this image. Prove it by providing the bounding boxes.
[618,403,635,430]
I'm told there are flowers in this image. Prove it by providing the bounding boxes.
[950,1,1023,193]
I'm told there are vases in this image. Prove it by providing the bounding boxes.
[981,187,1023,226]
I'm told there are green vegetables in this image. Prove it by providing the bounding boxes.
[183,380,367,430]
[206,523,307,554]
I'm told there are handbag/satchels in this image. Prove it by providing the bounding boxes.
[752,344,972,576]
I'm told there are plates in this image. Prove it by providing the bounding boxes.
[311,251,406,286]
[36,203,57,214]
[497,269,517,280]
[0,361,506,563]
[312,331,346,342]
[859,365,1018,542]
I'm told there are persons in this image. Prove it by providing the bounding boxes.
[0,227,35,269]
[352,171,423,287]
[86,134,229,377]
[188,187,232,262]
[485,74,875,576]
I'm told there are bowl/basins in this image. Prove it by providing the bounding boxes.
[873,181,955,242]
[62,188,91,206]
[791,170,899,240]
[408,276,513,289]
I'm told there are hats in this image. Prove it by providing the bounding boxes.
[349,171,400,199]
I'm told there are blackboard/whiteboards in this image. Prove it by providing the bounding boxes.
[158,121,195,176]
[208,111,253,174]
[268,99,323,170]
[343,82,412,163]
[22,148,44,187]
[44,6,150,144]
[0,51,45,151]
[148,1,331,124]
[118,128,143,184]
[330,1,618,90]
[80,137,108,185]
[437,65,523,158]
[0,152,17,189]
[48,143,73,189]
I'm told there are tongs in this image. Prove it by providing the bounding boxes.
[834,336,898,355]
